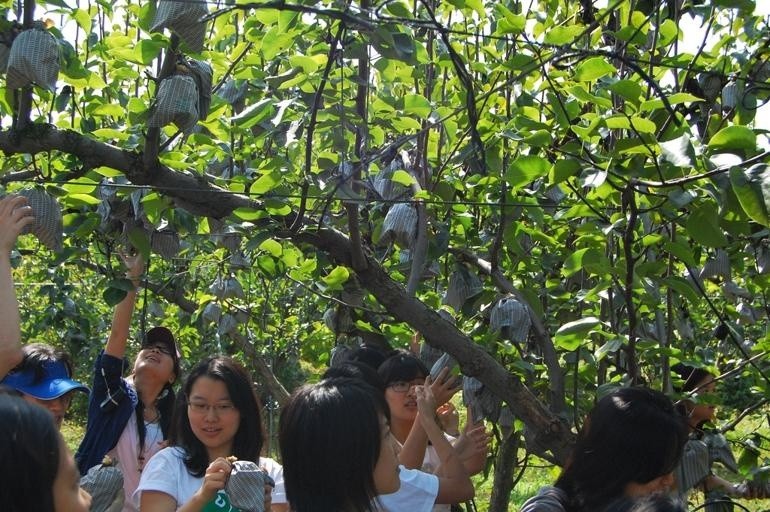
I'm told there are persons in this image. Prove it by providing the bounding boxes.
[666,365,768,502]
[132,353,291,512]
[273,328,491,511]
[68,240,191,512]
[518,385,689,512]
[0,394,97,511]
[596,488,688,511]
[1,339,126,511]
[1,192,42,384]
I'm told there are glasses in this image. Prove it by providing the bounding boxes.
[385,379,413,392]
[142,340,172,354]
[186,391,236,415]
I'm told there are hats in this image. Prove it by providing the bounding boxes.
[2,358,91,401]
[142,327,182,359]
